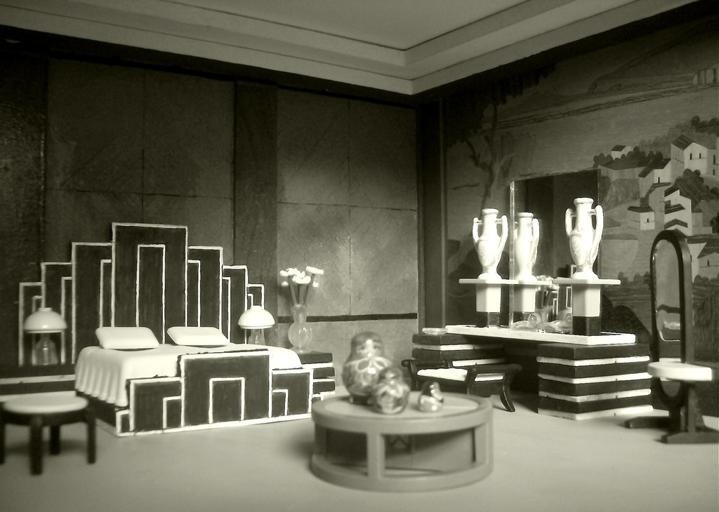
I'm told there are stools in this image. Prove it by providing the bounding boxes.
[0,394,96,475]
[402,356,521,412]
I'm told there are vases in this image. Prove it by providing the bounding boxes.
[472,208,509,279]
[288,306,312,354]
[565,197,604,279]
[513,212,540,281]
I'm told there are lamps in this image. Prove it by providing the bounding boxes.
[24,308,68,366]
[237,306,276,345]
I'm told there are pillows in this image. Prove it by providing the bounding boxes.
[167,326,229,348]
[95,326,159,350]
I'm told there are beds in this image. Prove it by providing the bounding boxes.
[18,224,314,437]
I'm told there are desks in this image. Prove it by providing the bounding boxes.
[308,392,495,493]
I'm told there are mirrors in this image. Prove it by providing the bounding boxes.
[649,229,695,409]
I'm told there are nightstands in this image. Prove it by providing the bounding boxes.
[0,363,76,397]
[298,351,336,402]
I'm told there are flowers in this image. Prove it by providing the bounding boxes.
[279,265,324,306]
